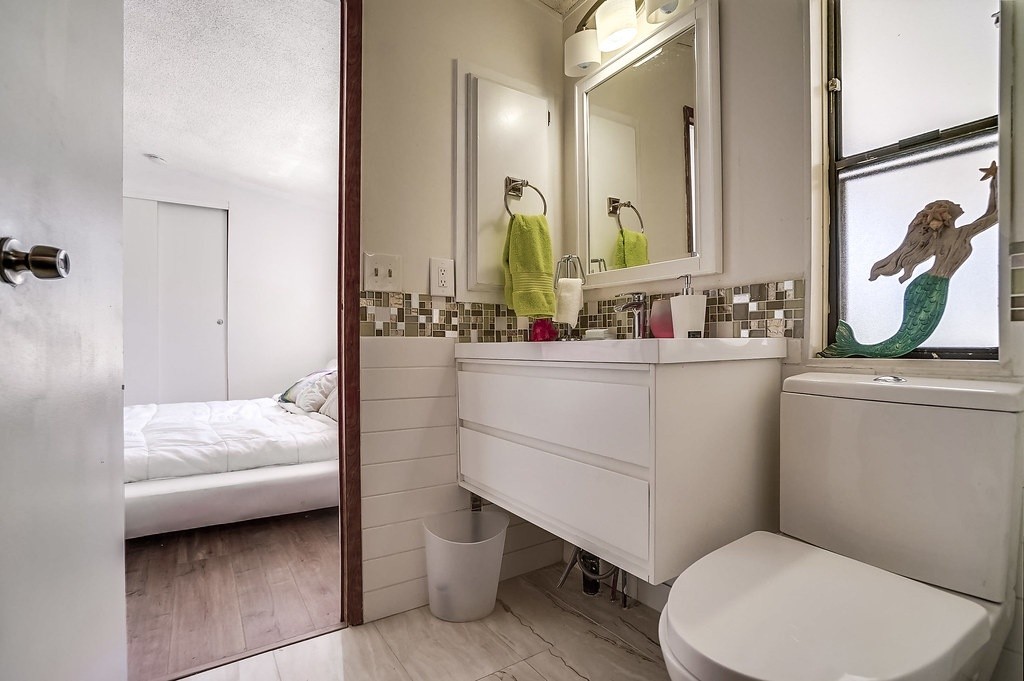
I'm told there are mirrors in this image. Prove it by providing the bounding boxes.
[581,19,702,276]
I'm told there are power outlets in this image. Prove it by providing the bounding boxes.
[429,258,454,297]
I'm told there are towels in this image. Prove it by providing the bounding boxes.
[555,278,583,328]
[614,228,649,268]
[502,214,556,319]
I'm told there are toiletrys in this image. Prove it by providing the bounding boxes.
[670,272,707,339]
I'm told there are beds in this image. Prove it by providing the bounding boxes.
[124,360,340,538]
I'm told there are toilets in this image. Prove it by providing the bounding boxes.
[658,373,1024,681]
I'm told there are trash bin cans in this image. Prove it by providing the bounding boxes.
[423,510,511,623]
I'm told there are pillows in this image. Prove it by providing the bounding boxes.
[318,387,338,421]
[274,369,337,413]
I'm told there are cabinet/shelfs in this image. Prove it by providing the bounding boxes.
[456,359,781,585]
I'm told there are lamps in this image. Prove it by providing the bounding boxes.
[645,0,694,24]
[595,0,637,52]
[564,25,601,77]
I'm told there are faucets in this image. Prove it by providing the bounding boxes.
[614,291,646,339]
